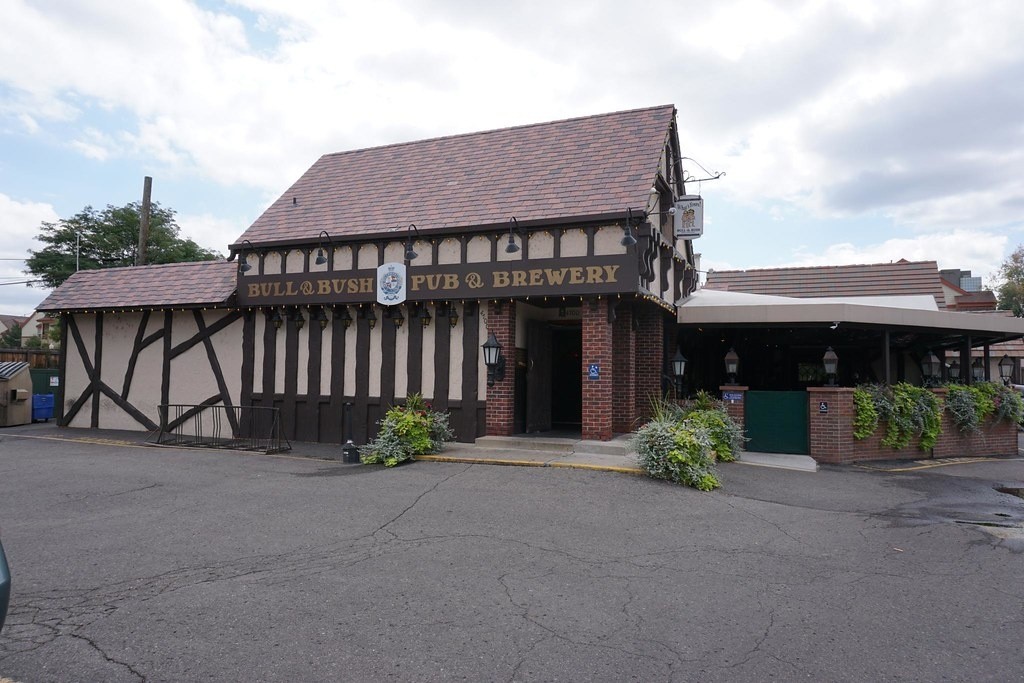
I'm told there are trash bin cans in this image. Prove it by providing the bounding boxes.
[0,361,33,427]
[32,392,54,422]
[30,369,59,418]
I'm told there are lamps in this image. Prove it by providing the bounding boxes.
[823,346,839,374]
[447,300,459,328]
[240,240,260,271]
[505,216,527,253]
[948,360,960,379]
[318,304,329,331]
[669,344,688,375]
[404,224,422,260]
[341,303,353,329]
[724,348,740,374]
[366,301,377,329]
[645,187,677,219]
[617,207,640,247]
[998,354,1014,384]
[272,307,282,331]
[293,305,305,331]
[481,329,505,388]
[972,356,984,378]
[391,305,404,329]
[418,301,432,328]
[315,231,335,264]
[919,347,941,378]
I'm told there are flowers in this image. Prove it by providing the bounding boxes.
[356,391,457,468]
